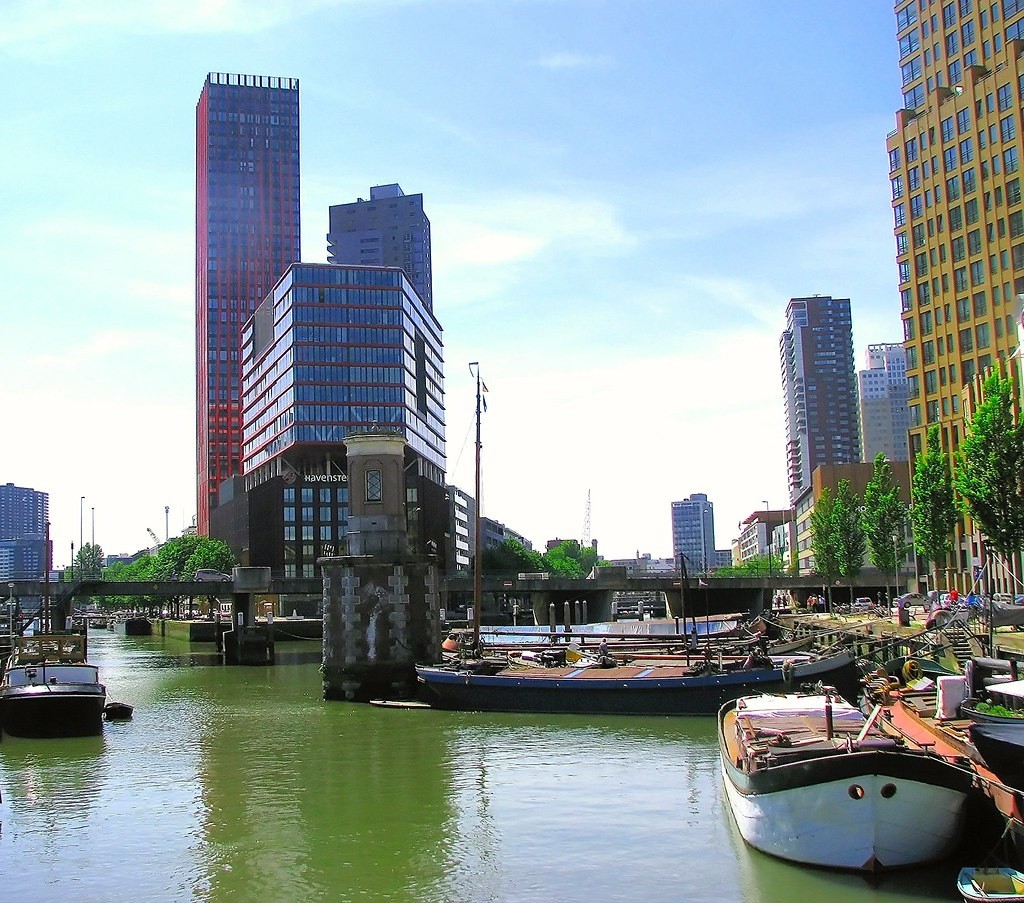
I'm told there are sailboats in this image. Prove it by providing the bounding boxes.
[0,520,107,731]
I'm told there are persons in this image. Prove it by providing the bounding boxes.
[773,593,826,613]
[948,587,958,605]
[599,639,608,657]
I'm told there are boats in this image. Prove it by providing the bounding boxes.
[414,360,865,713]
[858,656,1024,843]
[717,680,974,881]
[956,864,1024,903]
[104,701,134,722]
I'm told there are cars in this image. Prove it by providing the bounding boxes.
[854,596,873,610]
[928,587,1024,610]
[893,592,933,609]
[194,568,232,582]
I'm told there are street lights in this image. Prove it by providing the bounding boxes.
[80,496,85,582]
[892,533,899,598]
[763,500,773,577]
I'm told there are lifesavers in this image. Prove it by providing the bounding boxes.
[931,610,954,629]
[902,660,923,682]
